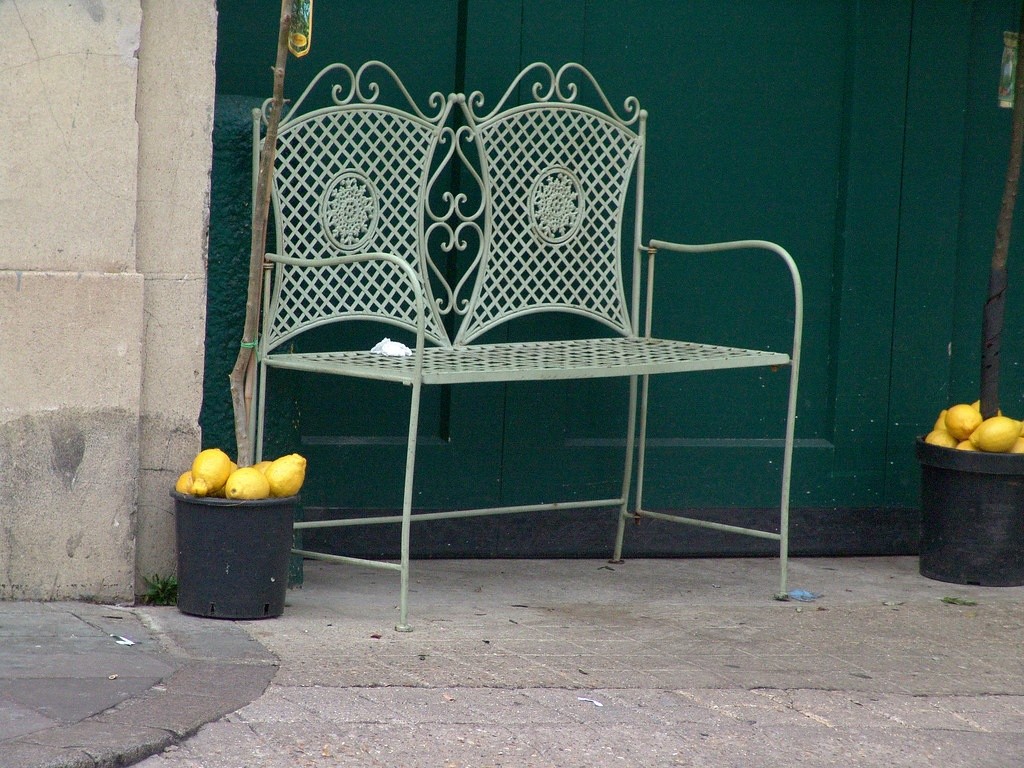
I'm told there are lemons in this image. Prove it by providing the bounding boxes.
[174,448,307,500]
[923,399,1024,454]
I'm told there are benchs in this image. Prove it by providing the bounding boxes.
[247,58,802,637]
